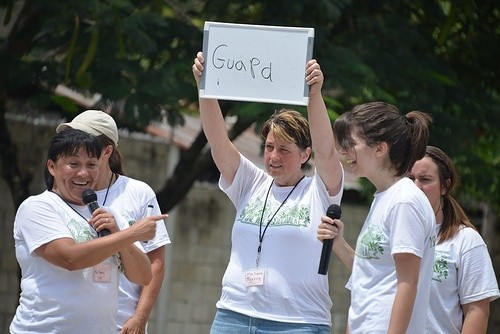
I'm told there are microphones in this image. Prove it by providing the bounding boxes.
[83,189,111,236]
[318,205,341,275]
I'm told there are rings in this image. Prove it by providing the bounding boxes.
[105,217,108,223]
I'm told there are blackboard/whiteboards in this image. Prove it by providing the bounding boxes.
[199,20,314,105]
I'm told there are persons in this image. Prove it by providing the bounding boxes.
[316,101,436,334]
[408,146,500,334]
[190,51,346,334]
[9,129,168,334]
[55,109,170,334]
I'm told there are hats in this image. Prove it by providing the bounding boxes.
[56,109,119,148]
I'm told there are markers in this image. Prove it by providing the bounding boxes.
[144,204,154,243]
